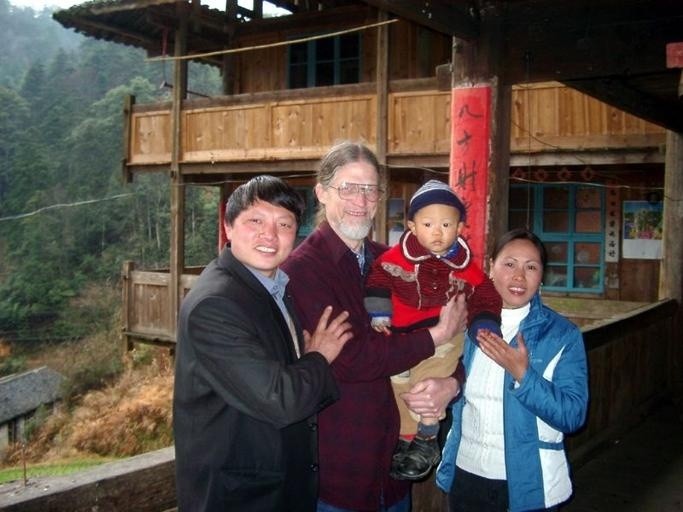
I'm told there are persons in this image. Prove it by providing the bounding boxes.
[362,179,503,481]
[434,227,589,511]
[279,142,468,512]
[172,174,354,512]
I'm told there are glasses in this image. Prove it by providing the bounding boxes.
[325,181,386,203]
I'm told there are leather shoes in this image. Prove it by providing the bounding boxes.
[390,438,410,479]
[397,437,442,481]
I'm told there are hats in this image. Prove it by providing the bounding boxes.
[409,179,466,221]
